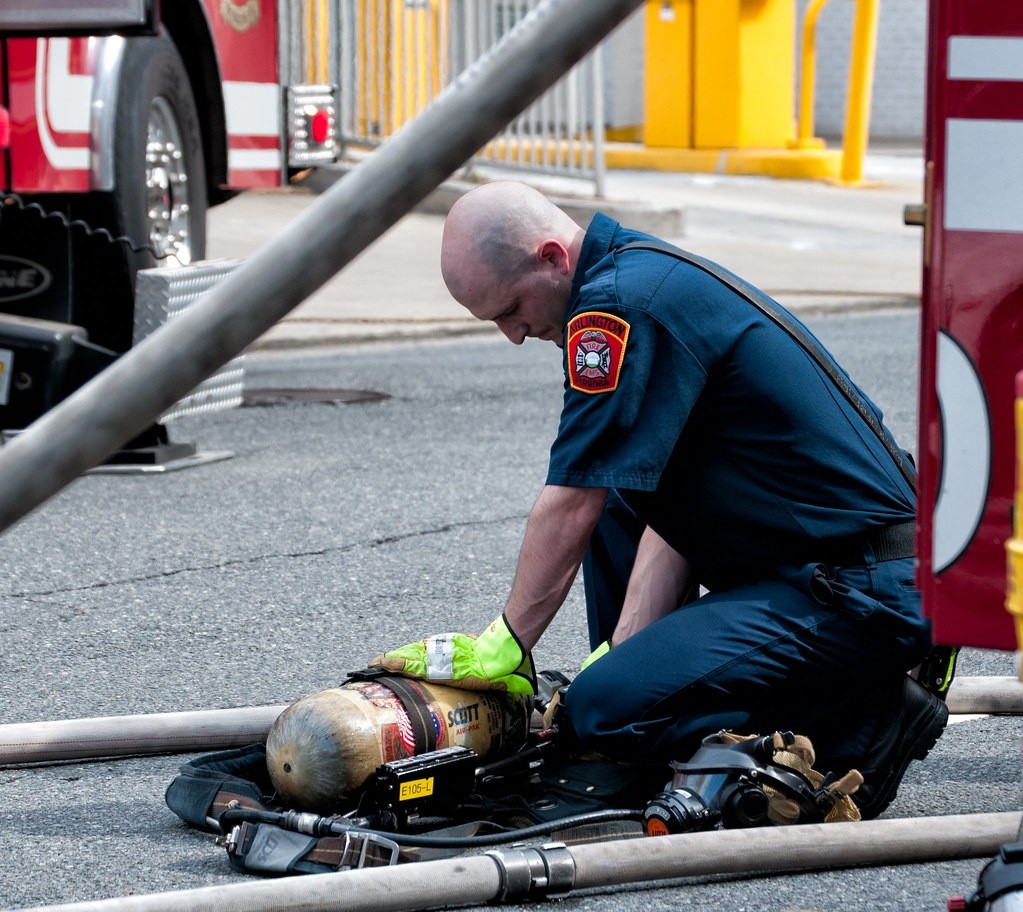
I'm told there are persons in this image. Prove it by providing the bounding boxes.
[370,181,958,820]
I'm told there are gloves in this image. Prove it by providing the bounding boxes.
[367,612,538,696]
[543,640,611,729]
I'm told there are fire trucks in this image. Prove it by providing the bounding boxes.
[0,1,343,478]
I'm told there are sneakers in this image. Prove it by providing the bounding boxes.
[847,675,949,821]
